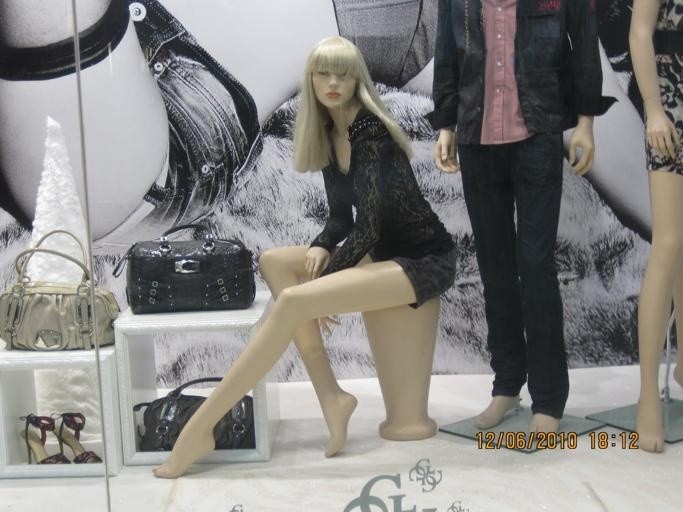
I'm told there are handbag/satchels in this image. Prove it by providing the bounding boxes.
[0,280,122,351]
[133,377,256,451]
[112,224,256,314]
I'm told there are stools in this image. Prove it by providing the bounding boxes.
[361,294,444,441]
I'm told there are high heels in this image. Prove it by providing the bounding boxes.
[19,413,103,464]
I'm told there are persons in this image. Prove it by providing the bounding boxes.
[151,35,458,479]
[627,1,683,455]
[0,0,656,243]
[430,1,618,442]
